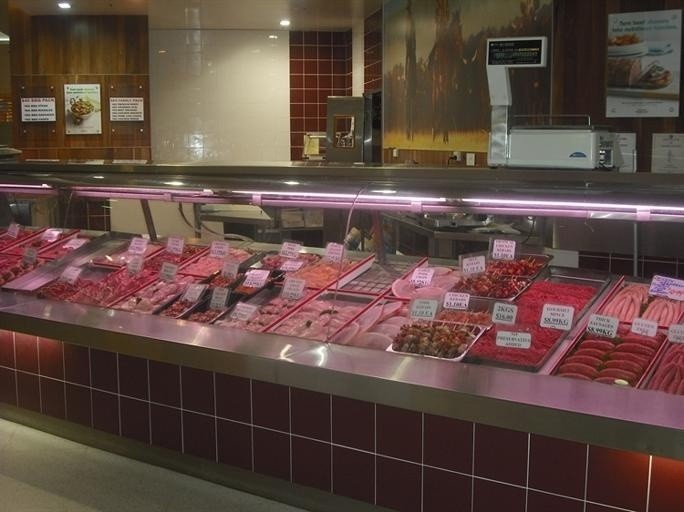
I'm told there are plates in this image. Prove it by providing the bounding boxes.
[648,41,673,55]
[608,39,648,59]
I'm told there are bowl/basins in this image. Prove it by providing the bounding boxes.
[69,100,96,119]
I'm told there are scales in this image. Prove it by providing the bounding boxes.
[485,37,624,170]
